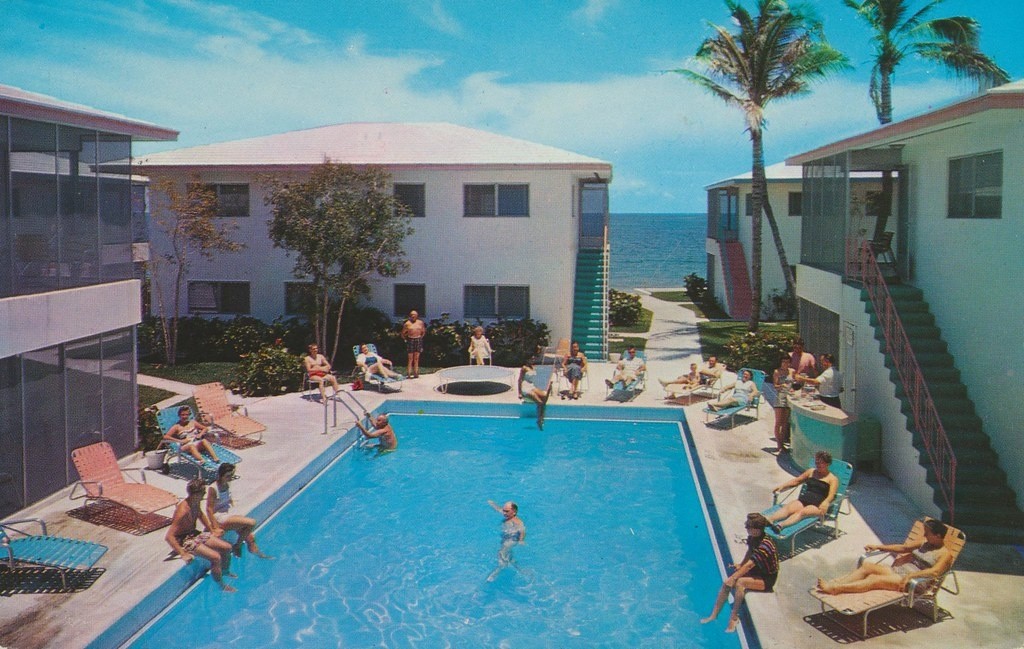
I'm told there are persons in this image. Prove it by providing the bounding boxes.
[354,409,398,460]
[786,337,819,391]
[355,342,402,381]
[303,342,342,407]
[400,310,426,379]
[205,462,275,560]
[162,405,222,466]
[681,363,701,390]
[772,354,798,453]
[700,512,780,634]
[164,476,239,593]
[817,518,952,595]
[657,355,722,386]
[485,499,527,583]
[517,351,547,432]
[560,341,588,399]
[467,325,492,366]
[605,344,647,388]
[760,450,840,534]
[706,369,759,412]
[794,352,845,410]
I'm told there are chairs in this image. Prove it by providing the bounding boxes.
[190,381,265,450]
[756,455,855,558]
[352,343,405,395]
[809,514,967,641]
[703,367,766,429]
[555,352,592,399]
[604,350,648,402]
[66,442,183,532]
[661,359,727,405]
[1,519,107,591]
[155,405,241,486]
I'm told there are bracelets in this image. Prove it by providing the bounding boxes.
[731,575,737,581]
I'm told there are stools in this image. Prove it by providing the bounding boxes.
[301,371,334,402]
